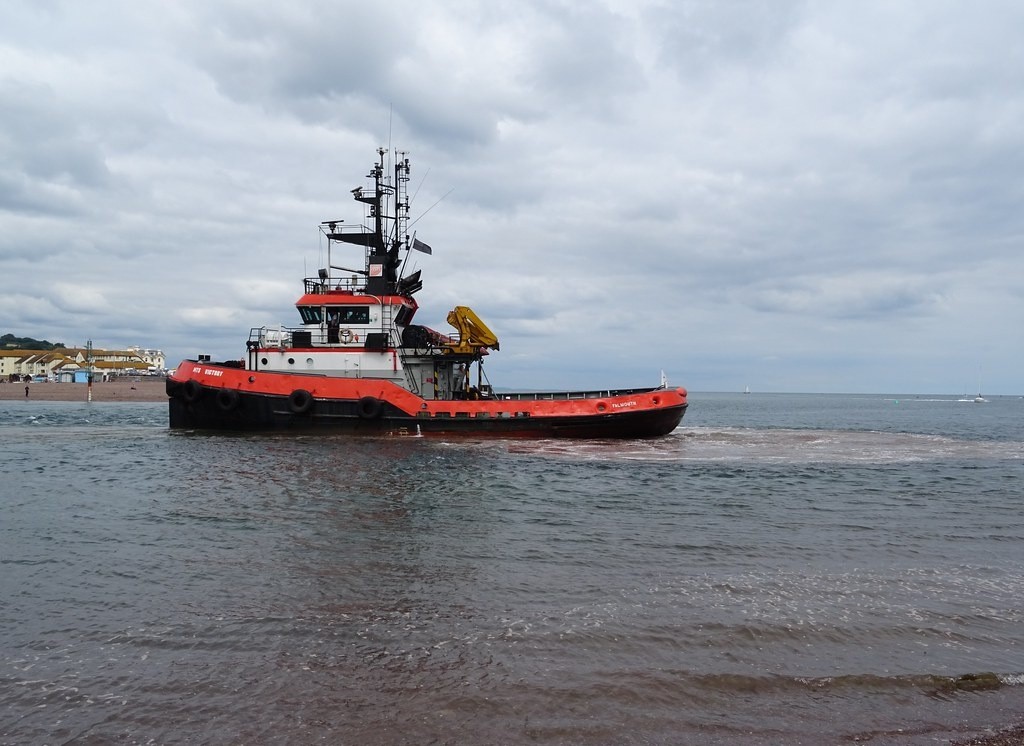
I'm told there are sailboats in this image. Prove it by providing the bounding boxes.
[744,387,750,394]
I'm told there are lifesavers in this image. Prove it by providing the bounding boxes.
[337,328,354,344]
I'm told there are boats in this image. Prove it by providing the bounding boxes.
[974,394,990,402]
[165,104,688,441]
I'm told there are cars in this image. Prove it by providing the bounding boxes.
[125,367,175,377]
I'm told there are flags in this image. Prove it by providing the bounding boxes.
[413,239,431,255]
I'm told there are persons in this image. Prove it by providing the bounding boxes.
[331,310,338,328]
